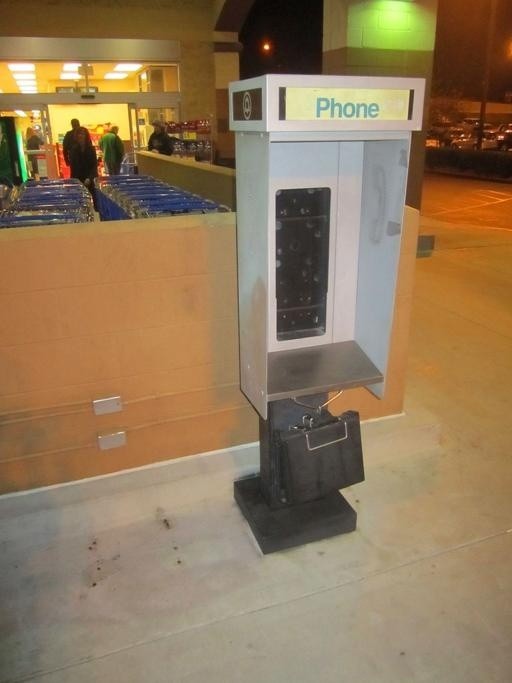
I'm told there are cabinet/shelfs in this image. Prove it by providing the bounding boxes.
[166,113,215,165]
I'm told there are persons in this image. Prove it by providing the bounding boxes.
[69,126,97,211]
[97,125,125,175]
[147,118,175,156]
[63,119,81,178]
[25,126,44,173]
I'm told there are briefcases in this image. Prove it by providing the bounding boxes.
[276,409,364,506]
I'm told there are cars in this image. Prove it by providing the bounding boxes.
[490,89,512,103]
[425,116,512,150]
